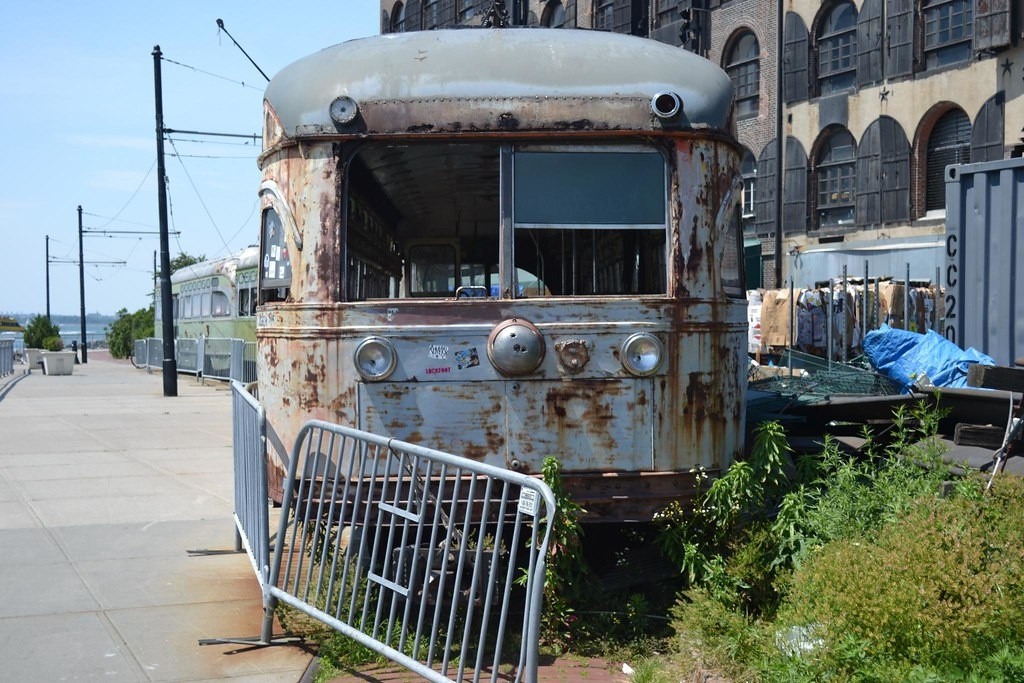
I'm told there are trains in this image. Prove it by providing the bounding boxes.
[155,24,749,581]
